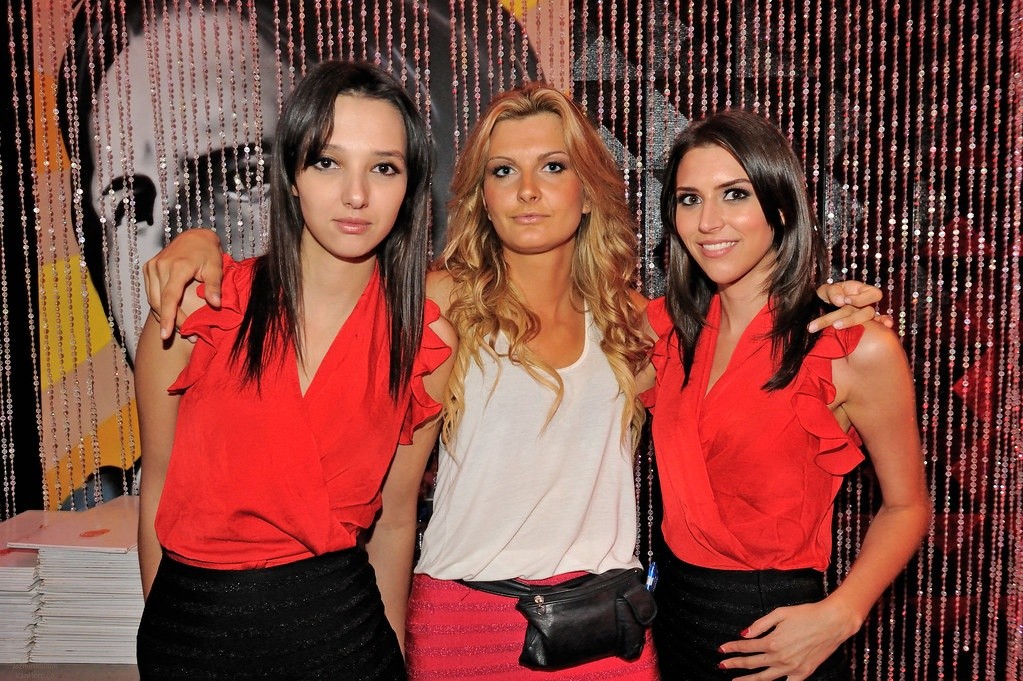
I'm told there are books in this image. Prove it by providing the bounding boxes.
[0,495,145,665]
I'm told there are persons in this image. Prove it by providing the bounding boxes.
[133,59,459,681]
[141,81,895,681]
[633,110,932,681]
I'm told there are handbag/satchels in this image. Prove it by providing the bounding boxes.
[455,566,656,671]
